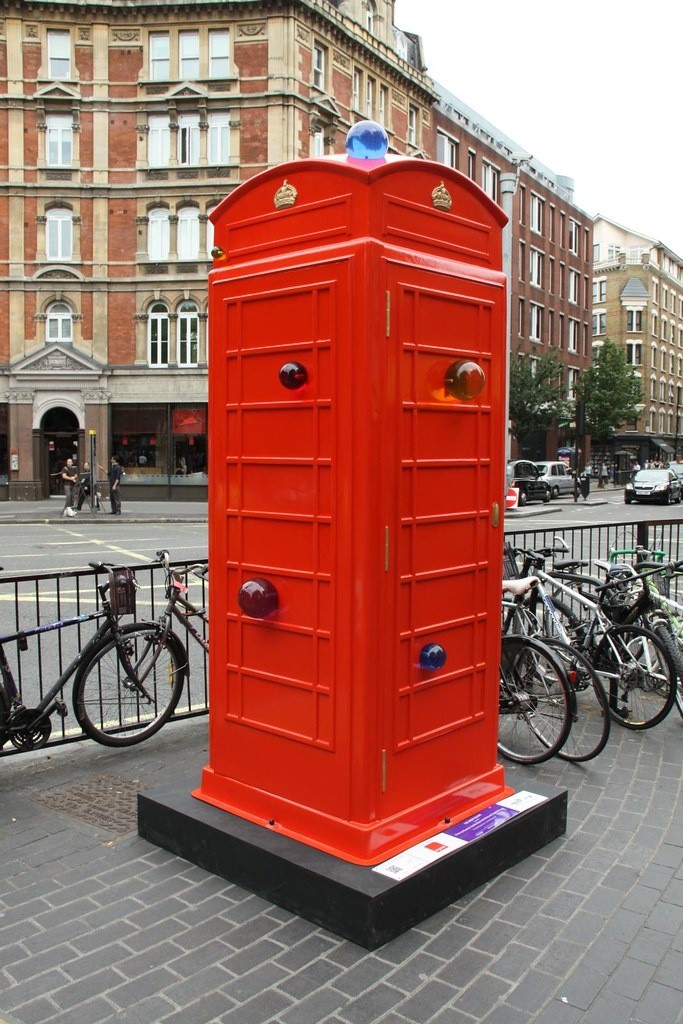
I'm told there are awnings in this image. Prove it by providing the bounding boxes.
[651,438,676,454]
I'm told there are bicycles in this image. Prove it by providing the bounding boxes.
[70,549,210,748]
[0,561,154,756]
[497,532,683,764]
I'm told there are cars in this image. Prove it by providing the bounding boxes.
[624,468,682,506]
[664,459,683,501]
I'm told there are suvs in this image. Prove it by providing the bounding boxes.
[532,460,581,500]
[506,459,552,507]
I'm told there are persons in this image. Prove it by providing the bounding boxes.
[585,460,683,489]
[176,457,187,476]
[96,455,123,515]
[62,458,79,517]
[73,461,101,511]
[115,450,155,468]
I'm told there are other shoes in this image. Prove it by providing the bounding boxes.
[108,510,116,514]
[116,510,121,515]
[75,508,81,511]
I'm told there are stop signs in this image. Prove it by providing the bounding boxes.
[504,487,520,511]
